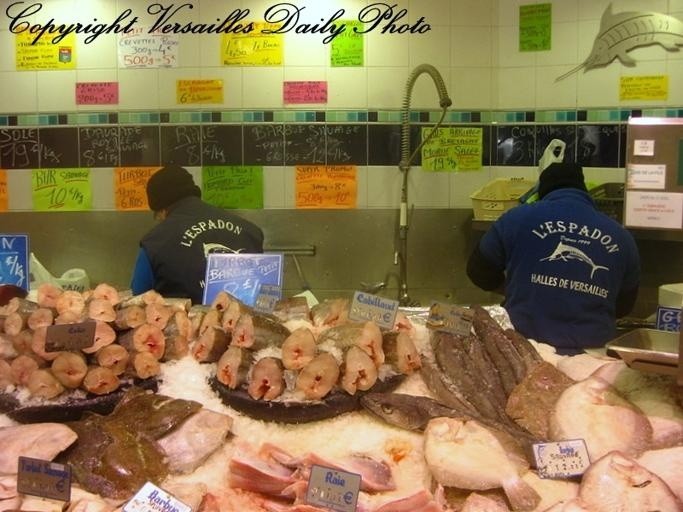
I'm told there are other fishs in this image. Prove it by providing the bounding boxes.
[359,303,681,512]
[51,389,235,500]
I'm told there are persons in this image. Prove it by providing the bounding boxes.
[130,164,266,307]
[462,161,641,356]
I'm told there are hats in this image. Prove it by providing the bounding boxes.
[145,165,200,211]
[538,162,588,199]
